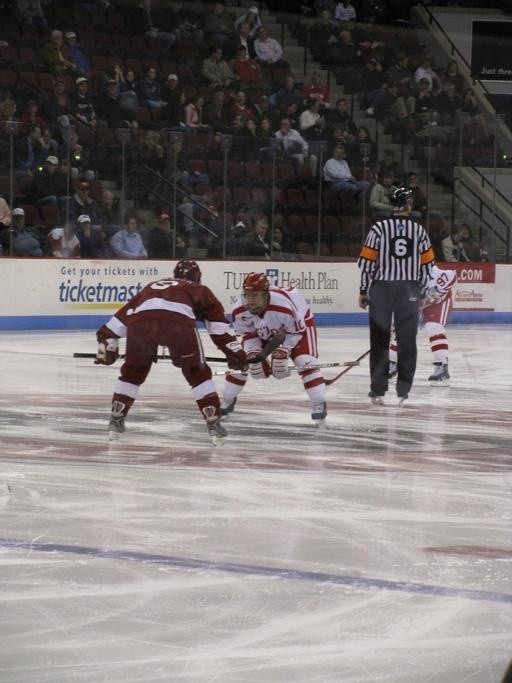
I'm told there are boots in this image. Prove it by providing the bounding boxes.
[202,406,228,439]
[428,357,451,382]
[311,401,327,420]
[388,361,398,379]
[107,400,127,433]
[220,398,236,415]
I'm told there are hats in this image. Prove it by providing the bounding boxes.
[167,74,177,81]
[106,79,118,87]
[419,77,429,85]
[45,156,58,165]
[77,215,91,224]
[11,207,26,217]
[158,214,170,224]
[74,77,88,86]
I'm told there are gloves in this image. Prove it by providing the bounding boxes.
[218,335,246,370]
[94,330,119,365]
[271,347,291,379]
[246,347,270,378]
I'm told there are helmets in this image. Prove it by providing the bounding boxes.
[241,272,270,316]
[389,186,415,207]
[174,259,201,282]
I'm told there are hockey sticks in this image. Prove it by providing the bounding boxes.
[74,330,285,363]
[323,330,396,384]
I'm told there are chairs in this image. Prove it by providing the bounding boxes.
[1,1,512,263]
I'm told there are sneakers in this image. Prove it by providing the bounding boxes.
[397,391,409,399]
[368,390,385,398]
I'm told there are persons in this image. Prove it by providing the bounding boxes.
[357,187,435,397]
[94,259,248,439]
[220,272,327,420]
[388,264,457,382]
